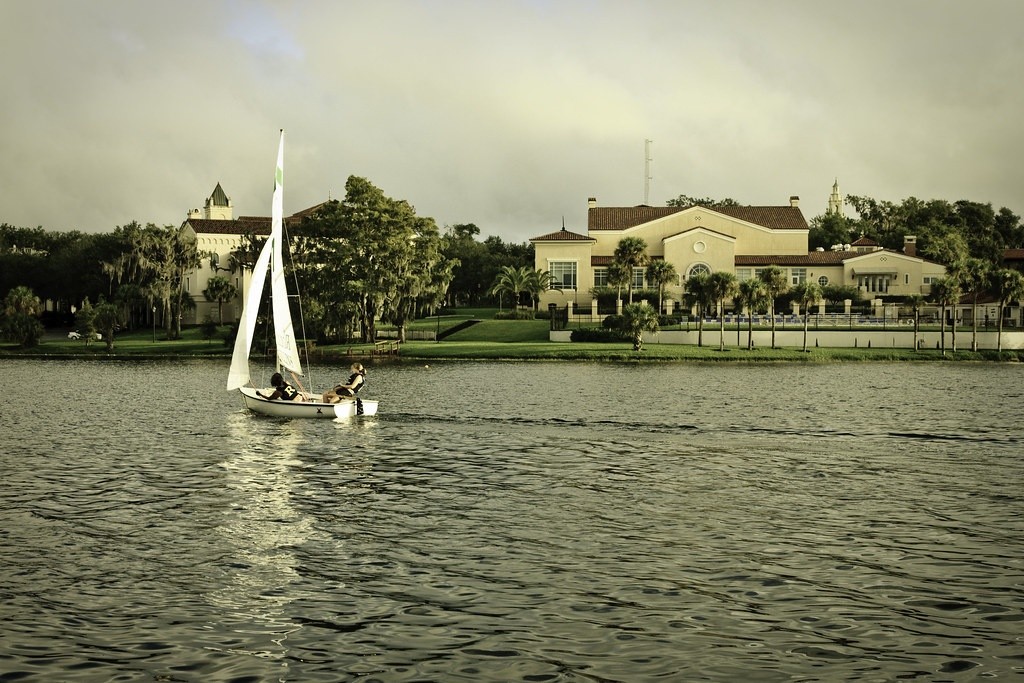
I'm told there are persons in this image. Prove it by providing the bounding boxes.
[256,372,305,401]
[323,363,366,403]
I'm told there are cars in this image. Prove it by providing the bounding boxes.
[68,331,102,341]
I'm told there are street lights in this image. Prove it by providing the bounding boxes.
[437,304,441,343]
[152,306,156,343]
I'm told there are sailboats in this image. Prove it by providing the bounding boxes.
[227,128,379,418]
